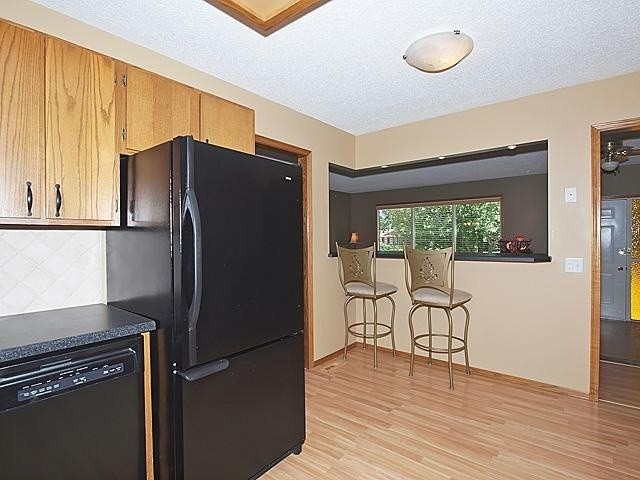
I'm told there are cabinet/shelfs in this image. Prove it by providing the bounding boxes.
[200,89,255,154]
[1,20,121,228]
[121,61,200,156]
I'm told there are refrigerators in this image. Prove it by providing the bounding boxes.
[106,135,306,479]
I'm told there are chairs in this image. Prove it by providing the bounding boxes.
[335,241,399,368]
[402,242,473,390]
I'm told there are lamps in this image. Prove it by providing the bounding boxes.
[404,31,474,72]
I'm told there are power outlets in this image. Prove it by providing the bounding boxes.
[564,257,584,273]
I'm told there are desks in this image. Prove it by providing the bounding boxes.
[0,303,157,480]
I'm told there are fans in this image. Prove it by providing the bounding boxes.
[600,143,640,174]
